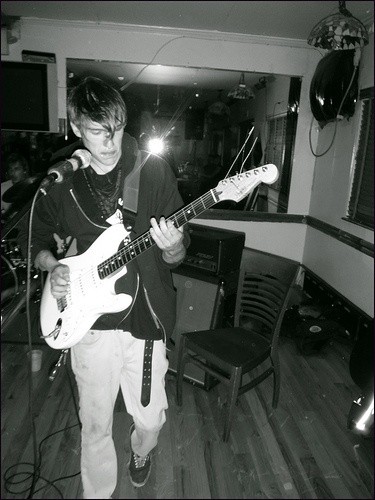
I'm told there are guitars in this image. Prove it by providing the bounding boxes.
[38,125,280,350]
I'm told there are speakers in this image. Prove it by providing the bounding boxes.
[162,266,240,391]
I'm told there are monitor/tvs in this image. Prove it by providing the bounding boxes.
[0,61,50,133]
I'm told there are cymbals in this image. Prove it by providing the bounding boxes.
[2,174,45,204]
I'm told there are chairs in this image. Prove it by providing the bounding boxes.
[175,247,303,442]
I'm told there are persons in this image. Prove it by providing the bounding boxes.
[18,76,190,499]
[1,154,37,213]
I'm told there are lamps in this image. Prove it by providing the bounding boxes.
[306,0,369,55]
[227,73,255,100]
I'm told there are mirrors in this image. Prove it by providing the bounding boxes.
[65,58,303,213]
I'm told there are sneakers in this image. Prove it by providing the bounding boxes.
[127,423,152,487]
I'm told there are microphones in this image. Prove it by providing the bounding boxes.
[41,149,92,186]
